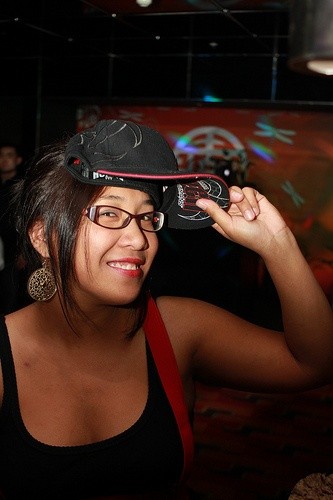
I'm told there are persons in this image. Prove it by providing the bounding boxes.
[0,138,31,315]
[0,120,333,500]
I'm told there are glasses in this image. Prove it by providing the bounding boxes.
[82,205,165,232]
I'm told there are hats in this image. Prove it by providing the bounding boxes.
[62,117,230,229]
